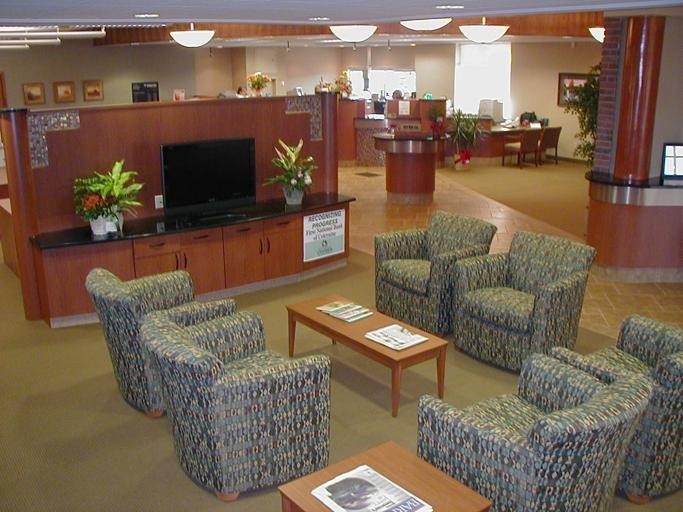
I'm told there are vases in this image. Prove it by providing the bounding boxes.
[88,213,118,234]
[283,186,304,206]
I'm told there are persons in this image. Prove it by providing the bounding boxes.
[314,298,375,325]
[362,324,431,352]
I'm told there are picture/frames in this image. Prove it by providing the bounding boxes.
[23,83,45,105]
[557,72,600,107]
[82,80,104,101]
[53,82,75,103]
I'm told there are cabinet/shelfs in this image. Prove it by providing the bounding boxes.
[29,234,134,327]
[129,227,226,311]
[299,196,355,286]
[221,211,301,298]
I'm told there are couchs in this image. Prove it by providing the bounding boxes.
[143,310,332,501]
[84,267,236,418]
[457,230,596,372]
[556,313,683,503]
[376,208,496,336]
[418,353,650,511]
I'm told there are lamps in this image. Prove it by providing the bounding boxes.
[458,16,510,44]
[400,19,452,33]
[331,24,377,50]
[588,25,604,41]
[168,21,215,49]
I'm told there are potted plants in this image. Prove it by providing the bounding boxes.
[520,112,536,127]
[427,103,444,140]
[448,109,489,170]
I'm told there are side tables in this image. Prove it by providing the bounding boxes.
[278,440,491,511]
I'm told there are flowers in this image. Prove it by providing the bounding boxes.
[264,135,318,187]
[248,71,269,90]
[70,158,145,219]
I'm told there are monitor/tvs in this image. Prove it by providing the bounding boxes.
[160,137,257,219]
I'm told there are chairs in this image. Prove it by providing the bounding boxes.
[537,126,562,165]
[502,129,541,168]
